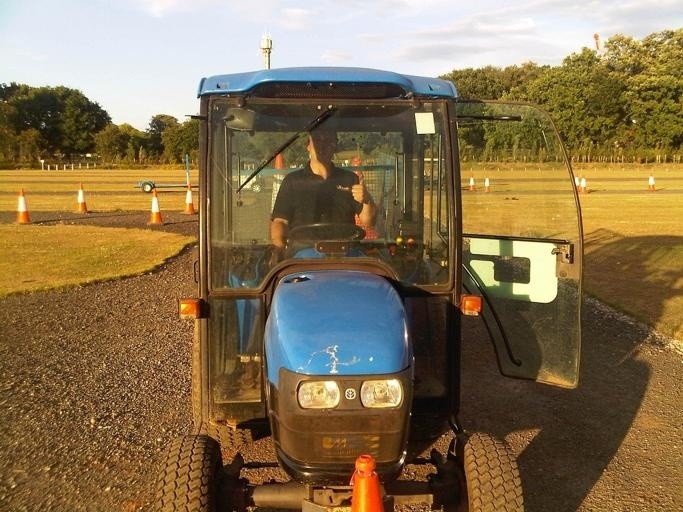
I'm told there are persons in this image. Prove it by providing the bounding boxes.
[268,128,377,253]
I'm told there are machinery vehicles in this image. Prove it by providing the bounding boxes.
[156,68,583,512]
[135,159,263,192]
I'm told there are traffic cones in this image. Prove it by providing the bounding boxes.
[468,175,476,191]
[484,177,490,193]
[647,173,656,192]
[73,182,89,212]
[13,188,33,226]
[180,184,196,215]
[575,177,586,194]
[147,188,164,224]
